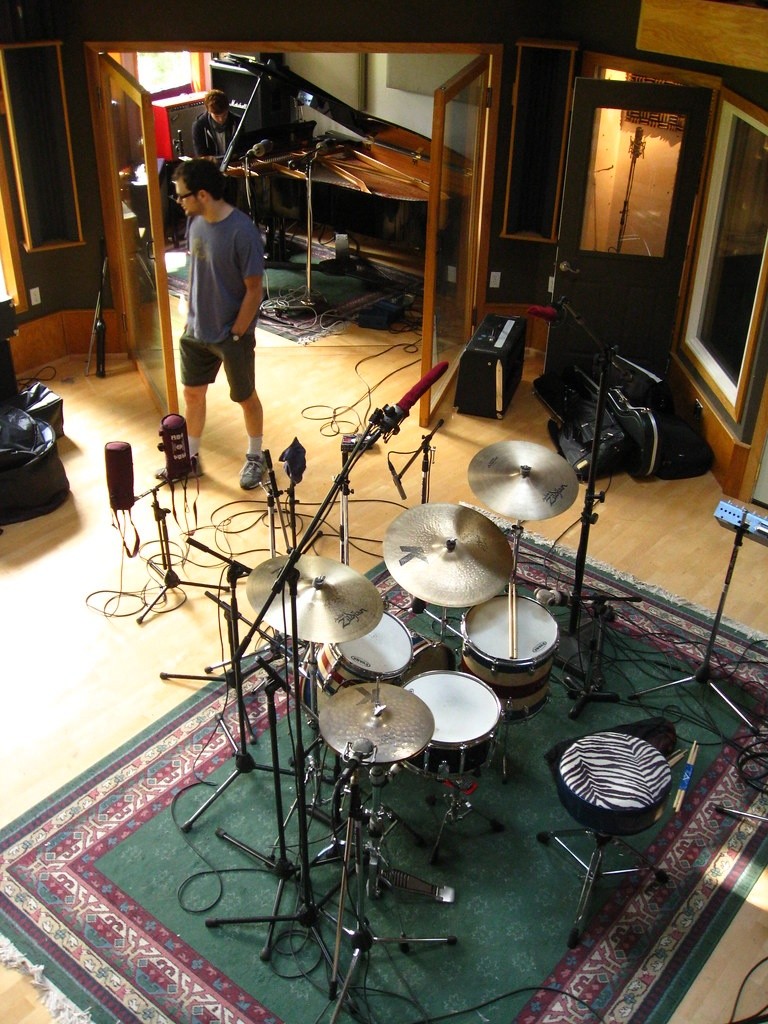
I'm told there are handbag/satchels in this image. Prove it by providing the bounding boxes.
[549,405,628,482]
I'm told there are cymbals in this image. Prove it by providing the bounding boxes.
[382,503,514,608]
[467,440,580,520]
[247,553,383,644]
[319,683,435,762]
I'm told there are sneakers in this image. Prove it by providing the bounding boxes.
[240,451,267,490]
[154,452,203,480]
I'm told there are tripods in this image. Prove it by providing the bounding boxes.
[137,490,230,624]
[627,526,760,735]
[159,563,457,1024]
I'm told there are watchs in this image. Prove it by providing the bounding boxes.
[230,331,242,342]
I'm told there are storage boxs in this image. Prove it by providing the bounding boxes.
[453,310,531,420]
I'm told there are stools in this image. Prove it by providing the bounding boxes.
[529,723,680,957]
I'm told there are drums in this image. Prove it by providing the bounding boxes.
[404,626,456,683]
[401,670,504,777]
[461,594,560,723]
[297,610,413,717]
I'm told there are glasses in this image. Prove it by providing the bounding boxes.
[176,189,198,199]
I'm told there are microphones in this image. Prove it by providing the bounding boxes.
[388,460,407,500]
[526,302,558,322]
[378,361,449,428]
[535,589,568,607]
[334,738,375,788]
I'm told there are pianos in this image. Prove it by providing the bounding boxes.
[161,53,474,262]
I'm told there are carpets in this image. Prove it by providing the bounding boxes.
[0,500,768,1024]
[164,231,422,345]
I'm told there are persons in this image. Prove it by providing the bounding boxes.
[152,157,268,490]
[190,91,247,205]
